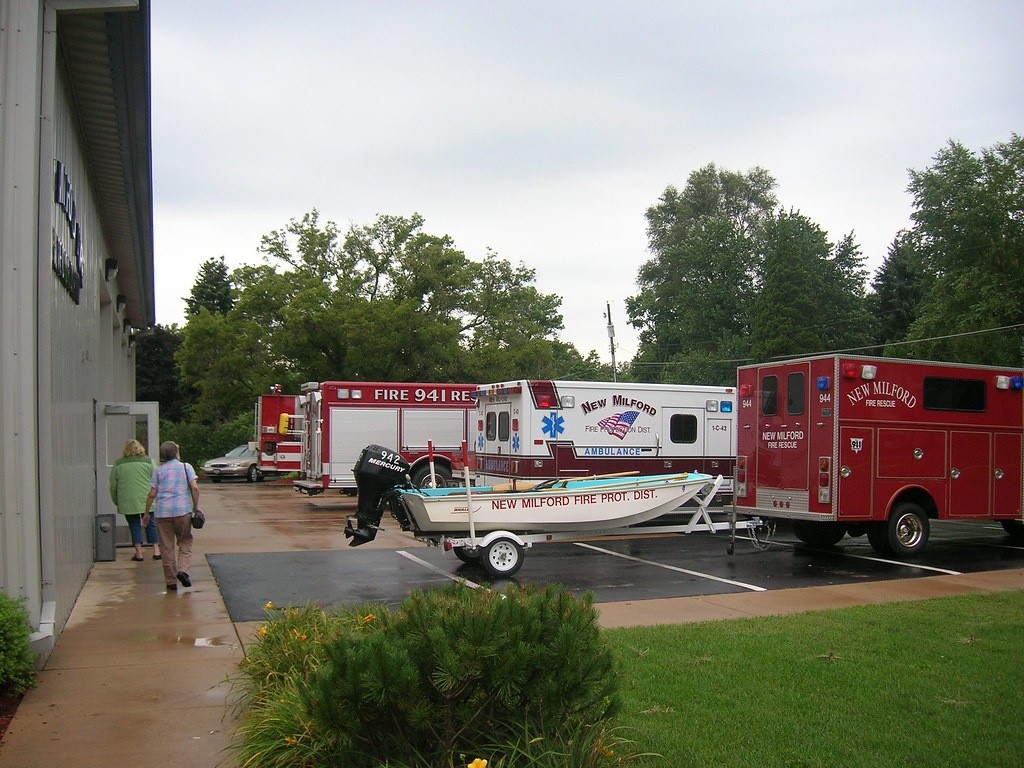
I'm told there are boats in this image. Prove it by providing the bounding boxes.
[343,444,714,548]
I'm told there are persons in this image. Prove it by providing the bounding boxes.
[143,441,203,590]
[110,439,161,561]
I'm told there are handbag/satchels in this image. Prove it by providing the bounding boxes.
[193,511,205,528]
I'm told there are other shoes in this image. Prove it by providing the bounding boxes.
[166,583,176,590]
[177,571,191,587]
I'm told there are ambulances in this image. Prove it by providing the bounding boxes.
[471,379,770,497]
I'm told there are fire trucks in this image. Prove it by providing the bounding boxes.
[733,352,1024,561]
[253,383,302,473]
[292,380,486,497]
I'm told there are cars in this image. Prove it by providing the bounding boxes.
[203,446,259,483]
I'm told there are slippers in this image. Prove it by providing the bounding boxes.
[132,554,144,561]
[154,552,162,559]
